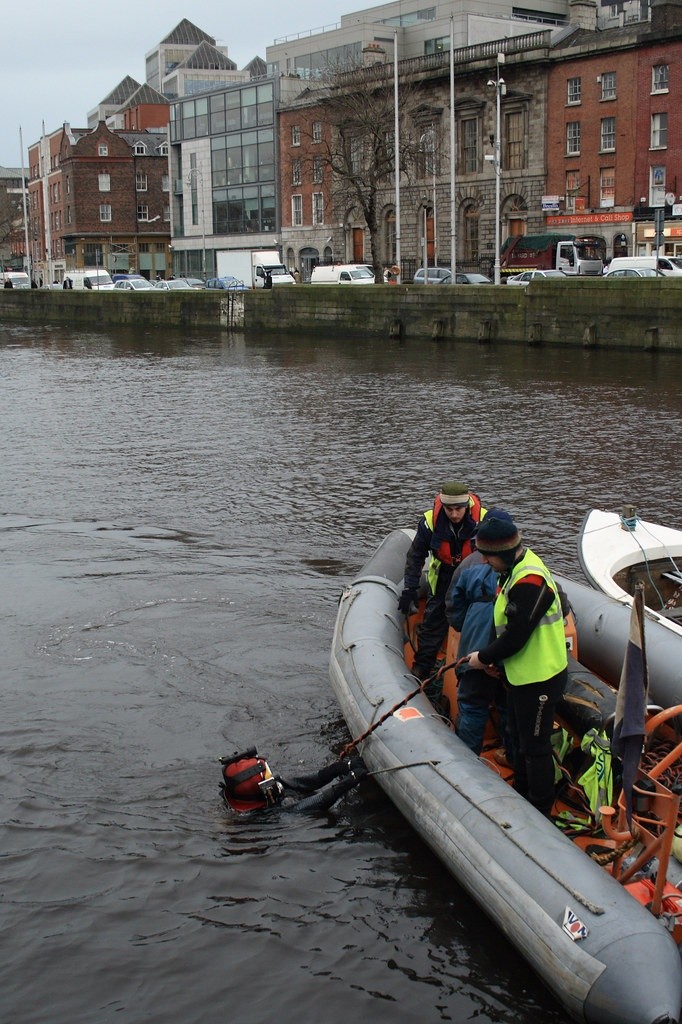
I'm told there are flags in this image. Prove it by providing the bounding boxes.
[610,590,648,838]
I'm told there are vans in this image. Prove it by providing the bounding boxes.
[311,264,381,285]
[600,256,682,277]
[413,267,466,285]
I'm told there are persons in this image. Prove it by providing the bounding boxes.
[467,518,568,819]
[397,481,487,682]
[31,280,37,288]
[444,508,515,757]
[4,278,13,288]
[85,278,92,289]
[383,267,389,277]
[63,277,72,289]
[156,274,161,280]
[167,274,176,280]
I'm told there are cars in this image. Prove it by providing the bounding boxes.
[0,268,204,290]
[507,269,568,285]
[205,276,250,290]
[437,273,495,286]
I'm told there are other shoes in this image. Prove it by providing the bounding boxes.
[411,667,425,679]
[494,749,513,769]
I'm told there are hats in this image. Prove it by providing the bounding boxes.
[441,481,470,507]
[482,509,513,522]
[474,517,521,593]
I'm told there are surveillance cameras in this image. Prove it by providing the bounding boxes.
[487,80,493,87]
[499,78,505,85]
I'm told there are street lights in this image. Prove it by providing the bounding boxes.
[486,53,507,285]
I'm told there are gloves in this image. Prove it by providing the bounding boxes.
[398,583,421,614]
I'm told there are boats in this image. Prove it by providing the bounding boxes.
[326,527,682,1024]
[577,506,682,639]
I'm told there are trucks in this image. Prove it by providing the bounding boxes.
[490,233,605,277]
[216,250,300,289]
